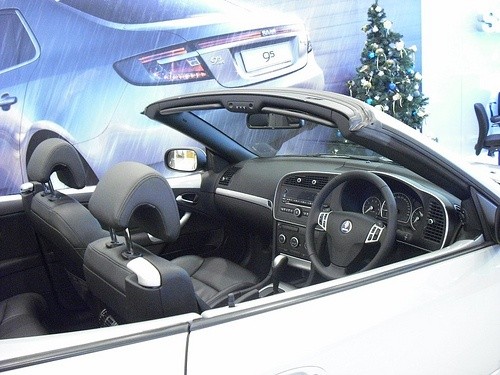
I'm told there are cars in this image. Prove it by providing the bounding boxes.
[0,1,325,203]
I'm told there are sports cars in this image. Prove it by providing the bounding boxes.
[0,91,500,373]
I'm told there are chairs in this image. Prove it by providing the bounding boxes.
[84,161,212,330]
[19,125,260,317]
[474,93,500,164]
